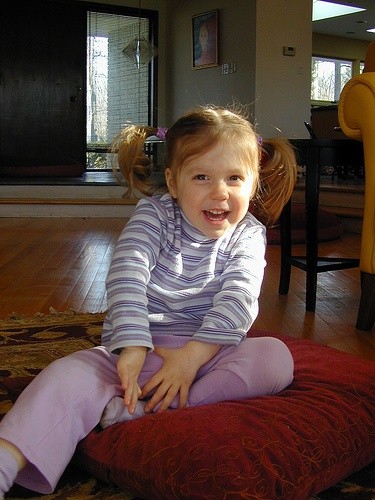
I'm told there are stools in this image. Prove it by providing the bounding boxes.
[278,136,365,313]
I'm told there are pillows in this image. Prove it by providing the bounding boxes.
[76,333,375,500]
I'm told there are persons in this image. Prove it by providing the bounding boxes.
[0,103,298,500]
[194,22,215,66]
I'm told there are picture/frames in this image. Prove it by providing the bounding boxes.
[191,9,219,72]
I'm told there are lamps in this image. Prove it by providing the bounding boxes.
[121,0,159,71]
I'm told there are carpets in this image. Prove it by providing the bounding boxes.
[1,307,375,500]
